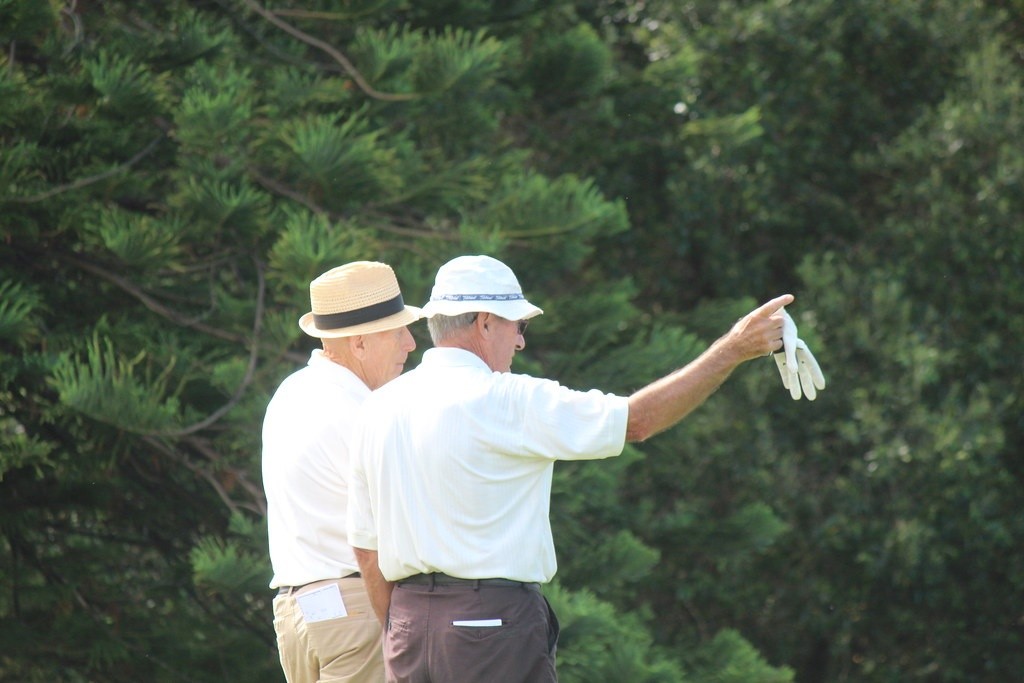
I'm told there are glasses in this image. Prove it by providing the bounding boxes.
[515,320,529,335]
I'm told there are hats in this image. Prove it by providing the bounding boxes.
[420,254,545,321]
[298,260,423,339]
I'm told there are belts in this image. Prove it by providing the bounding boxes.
[395,572,545,593]
[278,571,362,595]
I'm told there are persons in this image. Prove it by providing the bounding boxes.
[262,262,425,683]
[346,255,794,683]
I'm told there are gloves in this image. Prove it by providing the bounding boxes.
[769,307,826,402]
